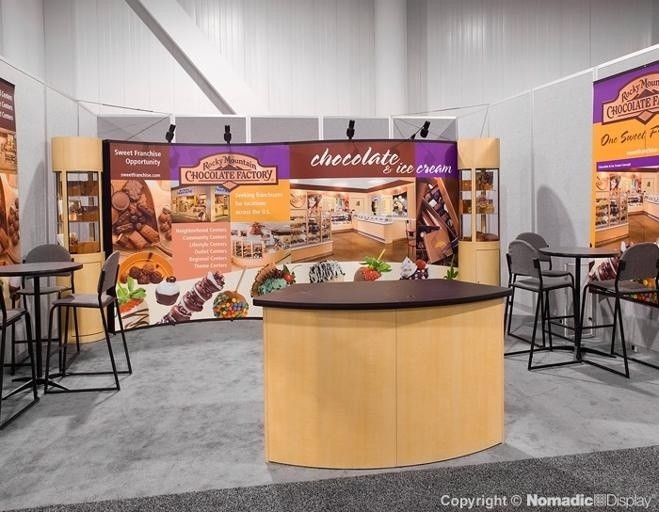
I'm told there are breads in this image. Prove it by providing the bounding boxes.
[470,231,499,241]
[462,200,495,214]
[460,180,492,191]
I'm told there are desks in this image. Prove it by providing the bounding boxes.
[249,278,517,473]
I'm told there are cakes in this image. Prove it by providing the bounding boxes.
[399,257,429,280]
[310,259,346,283]
[251,262,296,298]
[589,241,634,282]
[155,270,225,324]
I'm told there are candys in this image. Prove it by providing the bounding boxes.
[630,277,657,303]
[213,266,249,318]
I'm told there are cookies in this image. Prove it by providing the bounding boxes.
[120,264,163,284]
[0,197,20,253]
[110,180,172,250]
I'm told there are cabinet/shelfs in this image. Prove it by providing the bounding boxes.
[175,192,229,221]
[231,234,292,269]
[291,208,335,263]
[330,211,353,233]
[351,214,406,244]
[49,137,106,346]
[455,137,501,288]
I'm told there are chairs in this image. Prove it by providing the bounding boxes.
[0,243,132,433]
[502,231,659,377]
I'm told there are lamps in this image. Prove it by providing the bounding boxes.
[347,120,355,140]
[223,124,233,145]
[407,120,431,141]
[166,124,177,143]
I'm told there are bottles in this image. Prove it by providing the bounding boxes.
[77,201,83,221]
[479,191,493,213]
[479,169,488,190]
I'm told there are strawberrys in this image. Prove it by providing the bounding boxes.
[353,257,391,281]
[114,276,146,314]
